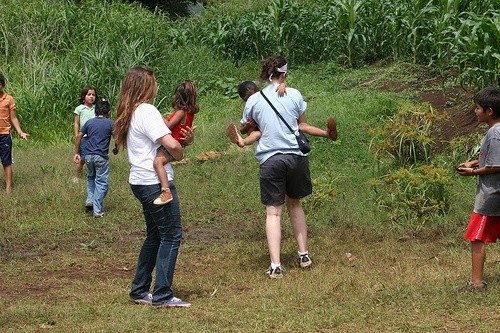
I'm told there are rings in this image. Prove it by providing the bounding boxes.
[185,136,189,139]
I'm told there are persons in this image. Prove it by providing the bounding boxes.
[73,101,115,218]
[455,87,500,292]
[225,80,338,148]
[74,87,100,184]
[112,66,198,307]
[152,79,199,205]
[239,56,312,279]
[0,74,30,194]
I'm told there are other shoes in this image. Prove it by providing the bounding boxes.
[73,177,80,183]
[327,117,338,141]
[227,123,239,143]
[94,212,104,218]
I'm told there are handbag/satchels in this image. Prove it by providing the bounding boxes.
[297,134,311,153]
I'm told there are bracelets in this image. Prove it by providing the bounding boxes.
[471,167,477,176]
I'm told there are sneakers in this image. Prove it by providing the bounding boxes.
[298,249,313,268]
[154,297,191,307]
[154,188,173,205]
[265,264,284,279]
[459,279,487,293]
[134,293,152,303]
